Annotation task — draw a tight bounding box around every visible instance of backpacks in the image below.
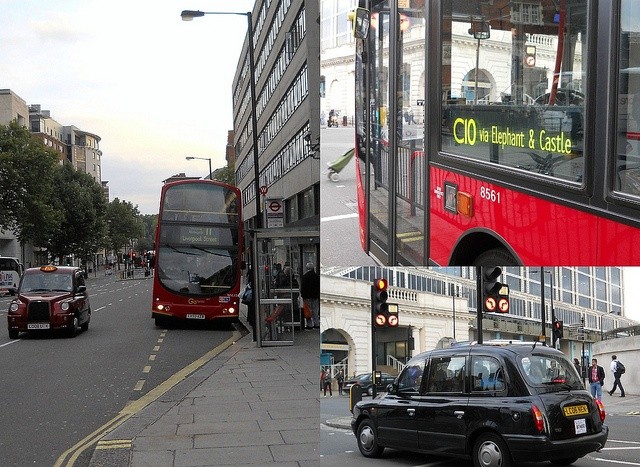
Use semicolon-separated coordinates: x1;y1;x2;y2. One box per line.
613;360;625;374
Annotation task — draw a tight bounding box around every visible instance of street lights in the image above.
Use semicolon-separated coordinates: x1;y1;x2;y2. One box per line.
185;155;213;183
180;10;259;219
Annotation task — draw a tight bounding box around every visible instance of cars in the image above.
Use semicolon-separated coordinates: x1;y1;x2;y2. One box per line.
343;371;397;393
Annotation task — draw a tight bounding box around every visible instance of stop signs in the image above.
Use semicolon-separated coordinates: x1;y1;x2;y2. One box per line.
268;199;285;214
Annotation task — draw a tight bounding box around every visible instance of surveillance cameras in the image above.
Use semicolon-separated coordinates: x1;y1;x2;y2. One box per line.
303;131;312;138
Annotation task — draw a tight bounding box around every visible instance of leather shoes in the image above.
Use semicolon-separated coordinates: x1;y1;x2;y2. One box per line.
619;395;625;397
607;390;611;395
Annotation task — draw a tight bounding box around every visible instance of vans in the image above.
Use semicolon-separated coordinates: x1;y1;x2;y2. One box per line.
0;255;23;292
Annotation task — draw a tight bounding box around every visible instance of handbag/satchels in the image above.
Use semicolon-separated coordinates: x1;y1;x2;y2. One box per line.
303;301;312;319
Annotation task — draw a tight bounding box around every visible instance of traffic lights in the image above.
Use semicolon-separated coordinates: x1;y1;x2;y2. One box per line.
555;320;563;338
370;278;390;313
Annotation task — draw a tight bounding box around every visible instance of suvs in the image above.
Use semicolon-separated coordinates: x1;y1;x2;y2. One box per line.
351;342;610;466
6;263;91;337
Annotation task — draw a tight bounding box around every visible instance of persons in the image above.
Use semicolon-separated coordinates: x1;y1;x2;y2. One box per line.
588;359;605;404
572;358;581;379
323;369;333;398
300;263;319;327
241;269;256;343
270;263;299;331
337;370;344;395
547;360;560;378
607;354;626;397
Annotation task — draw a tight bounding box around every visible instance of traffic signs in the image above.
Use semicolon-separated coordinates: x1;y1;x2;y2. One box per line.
372;313;399;327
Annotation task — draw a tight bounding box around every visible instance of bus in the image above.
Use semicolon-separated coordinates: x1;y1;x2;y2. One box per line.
151;176;244;325
347;1;640;264
